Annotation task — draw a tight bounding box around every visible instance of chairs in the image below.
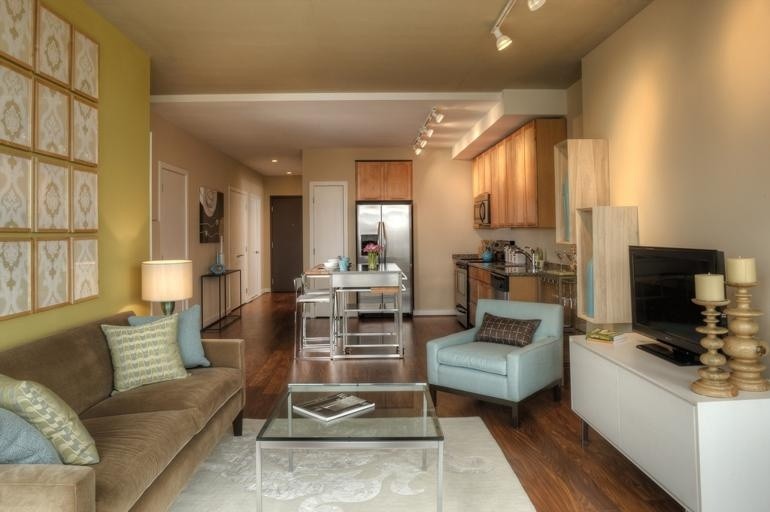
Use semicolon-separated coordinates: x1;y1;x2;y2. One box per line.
301;272;338;351
292;278;339;362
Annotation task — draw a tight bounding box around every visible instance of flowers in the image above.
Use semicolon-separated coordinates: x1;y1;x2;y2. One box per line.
362;242;385;269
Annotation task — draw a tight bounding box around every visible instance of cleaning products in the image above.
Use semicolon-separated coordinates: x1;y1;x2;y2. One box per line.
531;247;544;273
504;244;526;265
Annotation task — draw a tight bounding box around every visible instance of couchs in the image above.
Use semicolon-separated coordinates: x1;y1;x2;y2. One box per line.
425;295;567;427
0;311;248;512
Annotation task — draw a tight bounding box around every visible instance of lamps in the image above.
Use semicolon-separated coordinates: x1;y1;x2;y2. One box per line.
411;104;444;158
141;259;193;318
489;0;547;50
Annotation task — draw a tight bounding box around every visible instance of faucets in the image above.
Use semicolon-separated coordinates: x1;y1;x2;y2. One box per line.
513;247;531;263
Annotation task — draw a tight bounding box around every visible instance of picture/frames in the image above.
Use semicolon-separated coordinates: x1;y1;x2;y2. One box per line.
0;0;99;323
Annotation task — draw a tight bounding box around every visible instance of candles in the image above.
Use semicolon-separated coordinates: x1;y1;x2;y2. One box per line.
725;255;758;283
695;273;725;301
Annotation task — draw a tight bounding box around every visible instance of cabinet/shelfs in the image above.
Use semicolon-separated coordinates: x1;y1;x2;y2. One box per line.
466;264;490;325
567;328;770;512
471;116;567;229
355;160;413;203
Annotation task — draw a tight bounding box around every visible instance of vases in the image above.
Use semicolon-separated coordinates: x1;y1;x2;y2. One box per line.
368;252;377;269
218;236;226;271
210;249;224;274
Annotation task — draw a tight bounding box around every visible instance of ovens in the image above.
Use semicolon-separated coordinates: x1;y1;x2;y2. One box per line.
455;265;469;330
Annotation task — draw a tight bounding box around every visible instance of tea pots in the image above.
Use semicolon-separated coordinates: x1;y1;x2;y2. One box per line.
480;246;493;262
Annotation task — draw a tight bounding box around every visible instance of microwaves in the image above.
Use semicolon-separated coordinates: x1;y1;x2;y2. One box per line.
473;194;491;224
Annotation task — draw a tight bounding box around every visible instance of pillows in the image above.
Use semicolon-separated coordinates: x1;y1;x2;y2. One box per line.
101;312;191;398
474;308;542;348
1;408;64;467
126;302;210;368
1;370;101;466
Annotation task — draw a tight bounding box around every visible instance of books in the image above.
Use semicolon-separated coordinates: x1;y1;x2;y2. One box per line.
585;326;627;343
291;390;377;424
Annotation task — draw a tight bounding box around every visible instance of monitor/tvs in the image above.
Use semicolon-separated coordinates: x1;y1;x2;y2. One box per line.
629;246;728;367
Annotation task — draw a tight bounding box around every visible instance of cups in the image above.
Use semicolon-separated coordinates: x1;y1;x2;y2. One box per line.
323;255;350;271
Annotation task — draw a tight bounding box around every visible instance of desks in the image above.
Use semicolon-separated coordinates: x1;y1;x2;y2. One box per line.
200;268;242;332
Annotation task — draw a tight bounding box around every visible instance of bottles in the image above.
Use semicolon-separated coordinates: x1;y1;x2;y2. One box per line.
493;243;543;269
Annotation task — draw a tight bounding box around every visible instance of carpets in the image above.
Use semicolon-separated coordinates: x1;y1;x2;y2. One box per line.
172;414;536;511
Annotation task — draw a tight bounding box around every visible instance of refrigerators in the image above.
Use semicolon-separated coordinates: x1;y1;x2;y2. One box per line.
357;204;413;315
